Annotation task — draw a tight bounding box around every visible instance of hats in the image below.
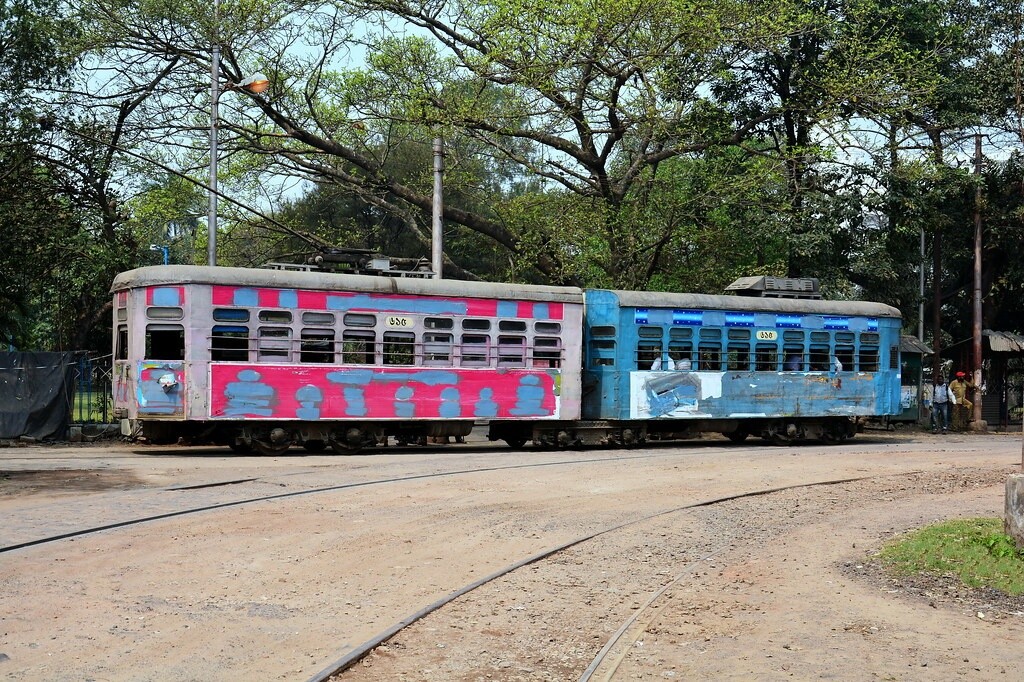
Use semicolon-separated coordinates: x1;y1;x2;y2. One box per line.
956;371;965;376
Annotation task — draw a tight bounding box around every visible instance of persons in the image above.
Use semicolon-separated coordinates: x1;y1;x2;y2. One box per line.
930;375;948;435
947;372;979;432
651;346;675;371
675;349;691;370
783;350;803;370
823;352;842;371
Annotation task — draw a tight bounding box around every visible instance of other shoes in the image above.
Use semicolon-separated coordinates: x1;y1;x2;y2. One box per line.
942;429;947;434
932;429;938;433
968;419;976;421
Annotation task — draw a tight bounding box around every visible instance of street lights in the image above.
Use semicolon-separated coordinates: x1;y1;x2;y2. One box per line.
208;42;271;265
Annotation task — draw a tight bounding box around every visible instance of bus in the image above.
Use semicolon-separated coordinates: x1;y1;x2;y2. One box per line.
36;117;902;457
